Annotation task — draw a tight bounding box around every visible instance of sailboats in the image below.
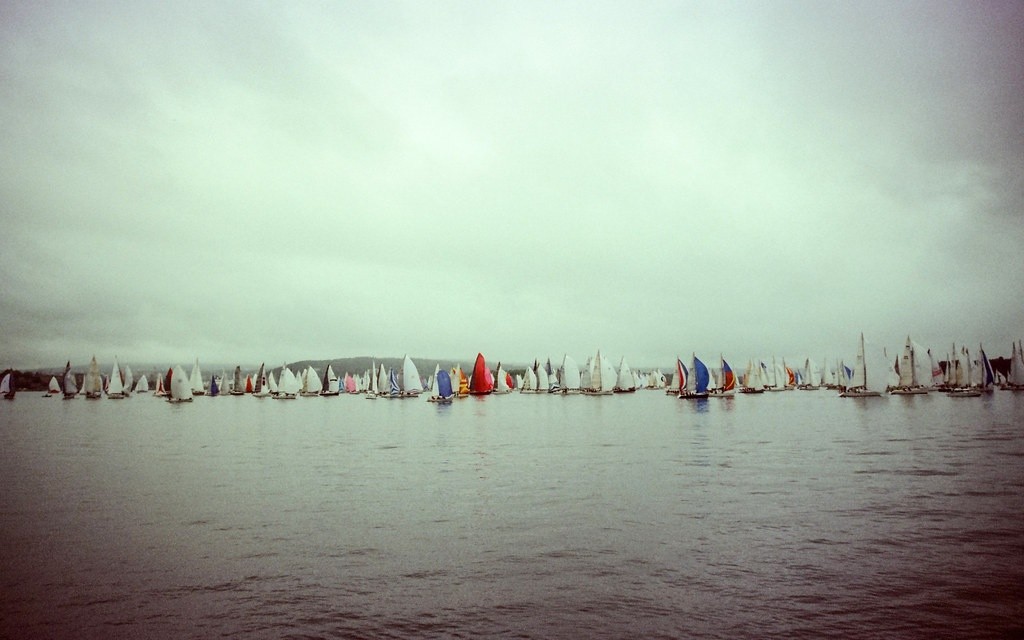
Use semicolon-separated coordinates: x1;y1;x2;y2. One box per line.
0;331;1024;412
